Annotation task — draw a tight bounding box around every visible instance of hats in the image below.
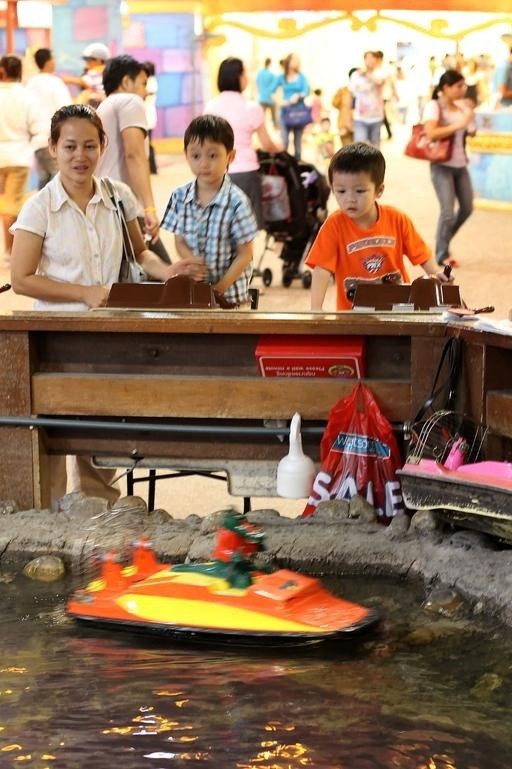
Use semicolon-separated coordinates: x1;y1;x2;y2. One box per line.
81;43;111;60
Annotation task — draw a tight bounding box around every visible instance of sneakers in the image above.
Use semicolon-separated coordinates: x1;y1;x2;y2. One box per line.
442;259;458;268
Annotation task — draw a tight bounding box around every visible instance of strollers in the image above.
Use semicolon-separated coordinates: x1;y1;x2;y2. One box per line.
248;143;333;290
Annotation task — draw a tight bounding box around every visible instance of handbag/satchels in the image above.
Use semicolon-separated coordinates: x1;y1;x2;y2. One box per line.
404;124;454;161
118;260;147;284
282;104;312;127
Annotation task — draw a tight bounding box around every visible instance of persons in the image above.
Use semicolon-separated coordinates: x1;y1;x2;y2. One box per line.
157;112;259;304
0;48;511;269
6;104;217;508
304;143;456;314
